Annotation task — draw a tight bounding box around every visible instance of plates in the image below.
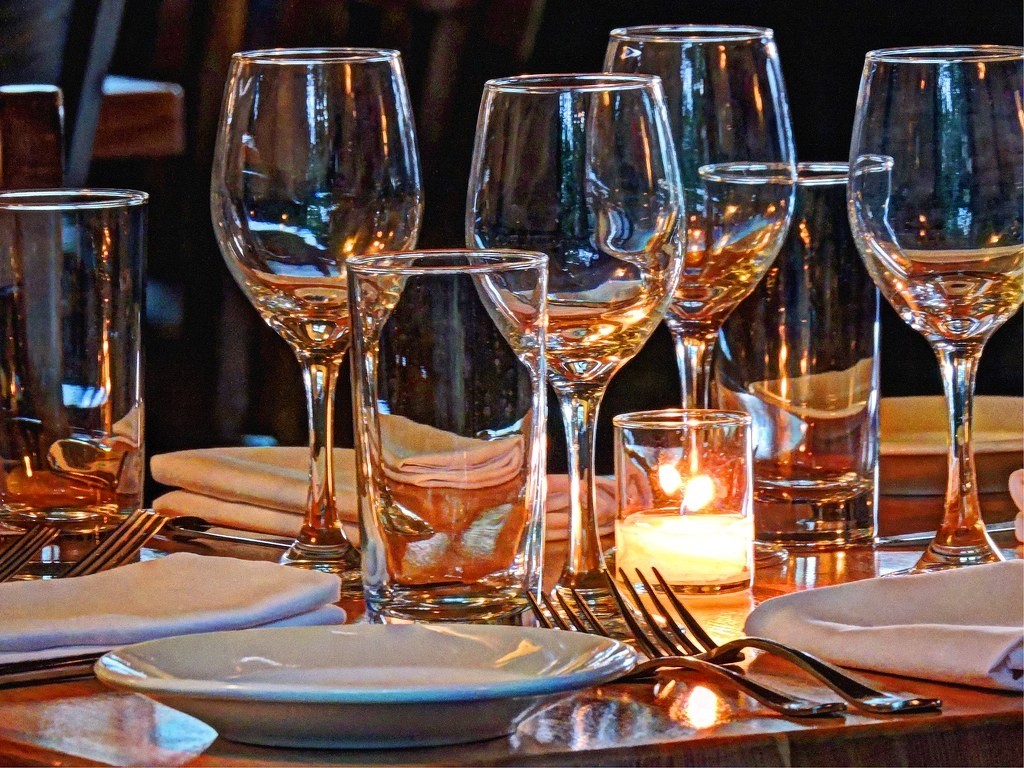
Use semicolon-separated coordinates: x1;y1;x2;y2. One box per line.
93;620;637;750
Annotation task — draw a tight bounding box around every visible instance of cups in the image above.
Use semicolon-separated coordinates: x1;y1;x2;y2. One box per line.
701;161;890;552
344;248;548;624
0;189;150;546
612;408;757;609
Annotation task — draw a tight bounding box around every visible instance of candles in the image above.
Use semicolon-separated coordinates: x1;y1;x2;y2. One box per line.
616;477;751;582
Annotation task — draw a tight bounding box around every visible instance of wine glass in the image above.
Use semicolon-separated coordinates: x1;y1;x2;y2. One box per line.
206;45;427;600
846;43;1024;578
585;18;797;404
462;73;687;615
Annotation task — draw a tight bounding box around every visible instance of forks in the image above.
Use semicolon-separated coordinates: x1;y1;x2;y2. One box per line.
602;565;943;714
526;586;848;714
63;508;170;579
0;523;61;584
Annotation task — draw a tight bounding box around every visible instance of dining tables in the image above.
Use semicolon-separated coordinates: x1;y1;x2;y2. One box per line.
1;495;1024;768
